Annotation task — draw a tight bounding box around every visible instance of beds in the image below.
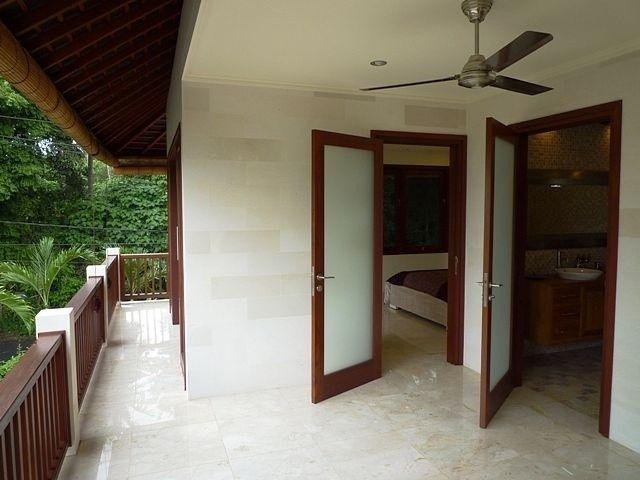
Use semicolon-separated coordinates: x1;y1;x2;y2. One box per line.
384;268;447;329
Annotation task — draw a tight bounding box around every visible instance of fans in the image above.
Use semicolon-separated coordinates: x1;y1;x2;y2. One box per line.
357;0;556;96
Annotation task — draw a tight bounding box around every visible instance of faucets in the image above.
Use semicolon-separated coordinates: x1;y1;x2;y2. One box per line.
556;250;565;267
576;257;593;267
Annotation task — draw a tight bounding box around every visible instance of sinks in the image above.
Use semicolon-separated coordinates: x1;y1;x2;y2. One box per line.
554;267;603;281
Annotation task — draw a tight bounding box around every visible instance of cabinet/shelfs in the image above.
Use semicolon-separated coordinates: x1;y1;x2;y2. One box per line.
582;284;603;340
549;287;580;341
